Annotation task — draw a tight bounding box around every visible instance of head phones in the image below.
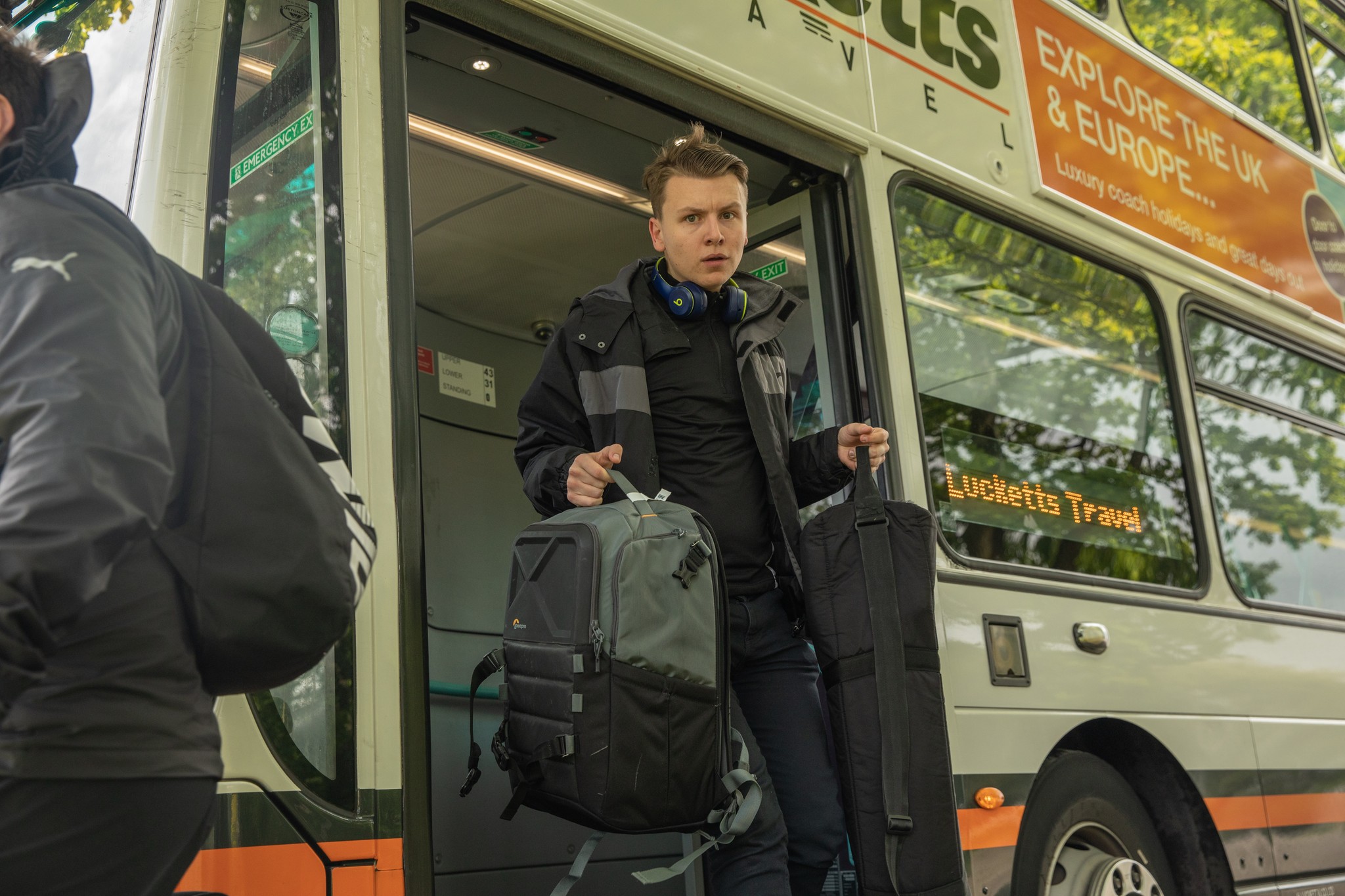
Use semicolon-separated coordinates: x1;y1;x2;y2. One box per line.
652;251;749;325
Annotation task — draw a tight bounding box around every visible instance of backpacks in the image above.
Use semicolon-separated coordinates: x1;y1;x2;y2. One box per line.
476;467;737;836
23;170;379;702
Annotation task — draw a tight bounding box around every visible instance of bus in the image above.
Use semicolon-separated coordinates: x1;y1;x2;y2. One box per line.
3;0;1345;896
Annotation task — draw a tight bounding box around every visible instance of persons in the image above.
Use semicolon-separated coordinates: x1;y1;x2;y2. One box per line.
0;25;225;896
515;121;891;896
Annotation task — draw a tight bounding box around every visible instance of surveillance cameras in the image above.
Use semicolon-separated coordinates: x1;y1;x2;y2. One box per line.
532;319;558;343
